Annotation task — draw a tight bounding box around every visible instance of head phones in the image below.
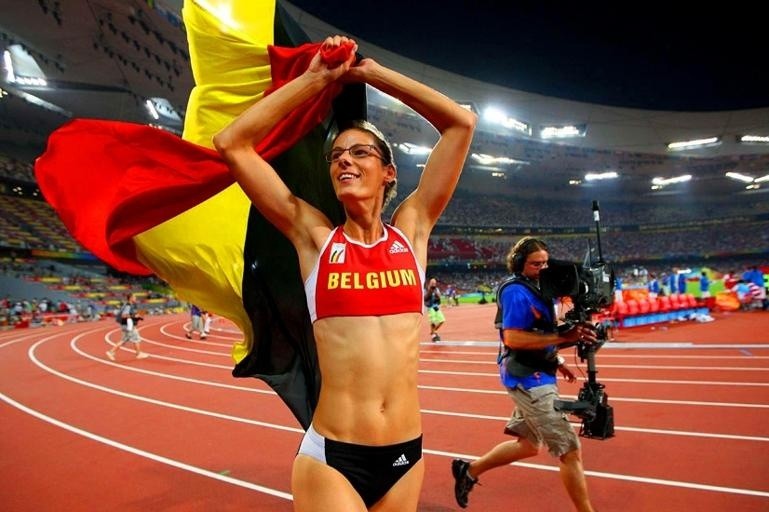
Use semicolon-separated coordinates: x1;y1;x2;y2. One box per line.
511;239;552;266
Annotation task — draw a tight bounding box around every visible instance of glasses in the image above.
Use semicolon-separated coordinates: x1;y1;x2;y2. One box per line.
326;142;386;159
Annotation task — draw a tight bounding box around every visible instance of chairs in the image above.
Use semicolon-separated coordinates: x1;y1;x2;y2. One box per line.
614;290;708;329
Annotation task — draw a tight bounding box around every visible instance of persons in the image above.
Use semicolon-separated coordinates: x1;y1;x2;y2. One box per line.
185;304;210;340
423;279;447;341
379;183;769;306
106;291;151;361
1;295;170;325
211;35;477;512
452;236;596;512
597;319;617;344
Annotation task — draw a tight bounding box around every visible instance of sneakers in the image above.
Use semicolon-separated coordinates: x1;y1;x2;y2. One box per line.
200;333;208;339
185;332;192;339
451;458;480;510
136;351;150;359
431;334;441;341
105;348;116;361
